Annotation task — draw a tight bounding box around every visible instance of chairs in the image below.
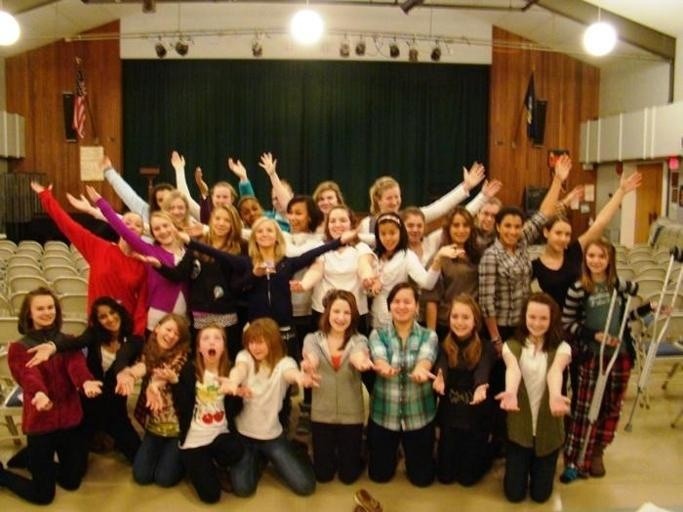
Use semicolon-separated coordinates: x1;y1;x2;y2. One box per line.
0;238;92;454
517;239;683;442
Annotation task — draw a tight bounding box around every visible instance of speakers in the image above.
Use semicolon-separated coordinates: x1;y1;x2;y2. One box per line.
63;94;77;142
525;186;548;245
527;100;547;146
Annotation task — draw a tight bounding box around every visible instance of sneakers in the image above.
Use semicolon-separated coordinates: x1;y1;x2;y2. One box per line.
591;456;604;476
8;446;29;469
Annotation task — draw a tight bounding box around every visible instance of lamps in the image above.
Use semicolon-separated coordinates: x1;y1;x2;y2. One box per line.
151;28;448;65
